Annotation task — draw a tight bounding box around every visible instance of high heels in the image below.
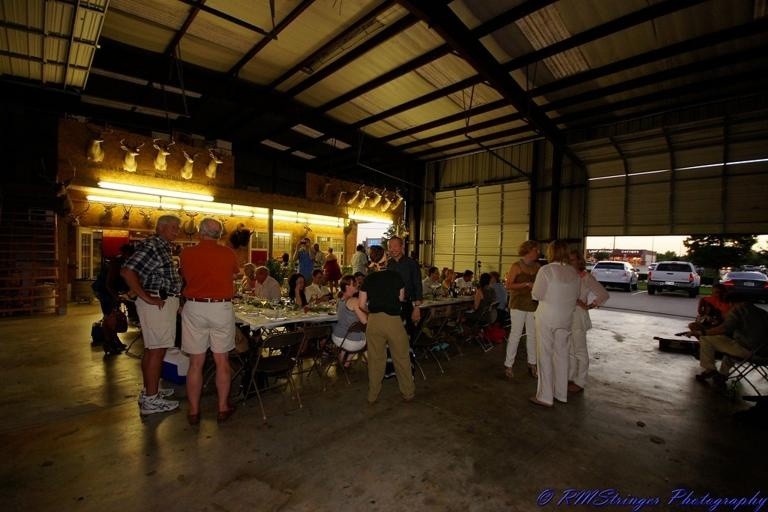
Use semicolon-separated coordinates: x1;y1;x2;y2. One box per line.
529;364;537;379
103;344;122;355
504;369;517;382
115;337;127;347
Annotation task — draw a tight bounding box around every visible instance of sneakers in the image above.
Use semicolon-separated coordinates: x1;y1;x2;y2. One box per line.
696;368;717;380
138;387;175;403
140;394;180;416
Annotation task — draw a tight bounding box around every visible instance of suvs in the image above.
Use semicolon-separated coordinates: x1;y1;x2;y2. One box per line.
647;261;705;300
591;260;640;291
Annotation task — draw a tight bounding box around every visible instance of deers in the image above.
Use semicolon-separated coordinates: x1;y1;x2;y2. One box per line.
205;149;224;179
180;144;199;180
119;137;146;173
320;175;332;195
82;127;114;163
152;137;175;171
333;183;404;213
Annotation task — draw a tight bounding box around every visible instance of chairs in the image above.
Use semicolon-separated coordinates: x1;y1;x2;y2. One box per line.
118;295;526;420
720;343;768;396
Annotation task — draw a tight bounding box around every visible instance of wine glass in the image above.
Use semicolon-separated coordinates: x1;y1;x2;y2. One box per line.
233;295;338;321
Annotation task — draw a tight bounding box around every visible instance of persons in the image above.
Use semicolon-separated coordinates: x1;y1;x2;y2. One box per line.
118;214;185;418
684;283;767;381
530;236;582;409
357;244;418;406
178;217;241;426
567;247;611;394
89;235;511;384
501;240;543;379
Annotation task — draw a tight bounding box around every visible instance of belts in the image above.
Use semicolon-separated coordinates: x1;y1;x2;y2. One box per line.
148;290;181;298
188;298;231;303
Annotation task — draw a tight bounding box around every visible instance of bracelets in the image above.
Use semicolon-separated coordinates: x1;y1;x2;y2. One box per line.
589;303;593;309
525;281;530;286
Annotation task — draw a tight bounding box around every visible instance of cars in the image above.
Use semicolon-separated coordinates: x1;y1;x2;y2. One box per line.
712;272;767;306
648;261;657;270
586;261;595;270
721;264;766;272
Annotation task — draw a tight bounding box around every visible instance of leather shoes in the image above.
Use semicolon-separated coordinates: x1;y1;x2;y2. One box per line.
528;384;585;407
186;411;200;425
216;407;236;424
